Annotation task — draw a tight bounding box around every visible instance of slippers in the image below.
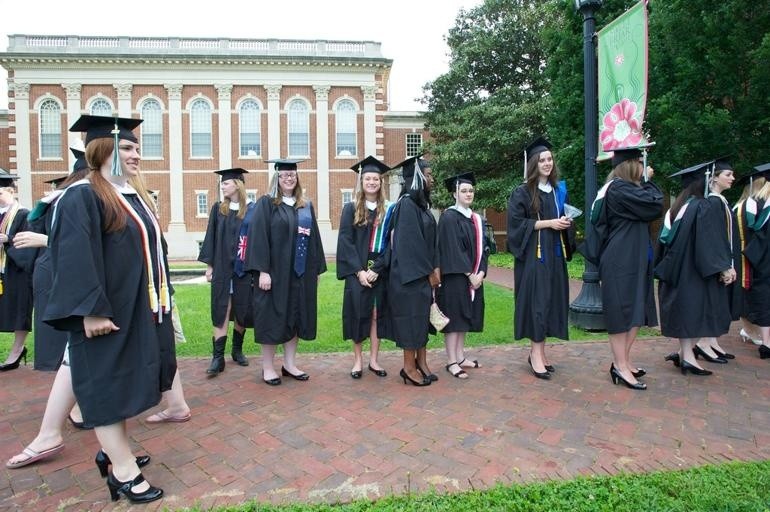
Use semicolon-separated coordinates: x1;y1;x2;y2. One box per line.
6;444;65;469
145;412;191;423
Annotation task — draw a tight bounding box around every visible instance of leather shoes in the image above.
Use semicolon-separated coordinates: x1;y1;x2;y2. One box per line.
68;415;94;429
369;363;387;377
282;366;310;380
350;371;362;379
528;355;554;379
262;369;281;385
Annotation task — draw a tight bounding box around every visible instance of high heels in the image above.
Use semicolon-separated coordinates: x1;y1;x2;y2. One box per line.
0;346;28;371
400;360;437;386
758;345;770;359
664;344;735;375
740;329;762;345
106;470;163;503
610;362;648;390
95;450;150;478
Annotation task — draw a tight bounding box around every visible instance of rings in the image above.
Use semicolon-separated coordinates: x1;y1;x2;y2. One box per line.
438;284;443;289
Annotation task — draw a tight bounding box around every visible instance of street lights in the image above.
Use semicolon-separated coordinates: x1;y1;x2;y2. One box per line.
569;0;652;333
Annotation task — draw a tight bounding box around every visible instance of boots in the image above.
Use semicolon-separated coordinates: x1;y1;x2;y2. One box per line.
206;335;227;375
231;328;250;366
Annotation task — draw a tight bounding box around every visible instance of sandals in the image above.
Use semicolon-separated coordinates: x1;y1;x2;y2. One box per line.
458;358;483;368
445;362;469;379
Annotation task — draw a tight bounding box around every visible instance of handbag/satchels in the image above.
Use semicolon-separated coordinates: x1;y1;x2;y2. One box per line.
430;304;450;331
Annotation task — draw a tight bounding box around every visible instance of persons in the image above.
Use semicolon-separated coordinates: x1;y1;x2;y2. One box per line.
376;165;444;387
244;170;329;387
198;179;256;376
505;150;574;380
588;146;770;392
437;183;489;380
1;115;193;505
336;172;394;380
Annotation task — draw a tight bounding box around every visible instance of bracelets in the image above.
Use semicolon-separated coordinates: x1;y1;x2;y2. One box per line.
538;221;541;230
467;272;474;279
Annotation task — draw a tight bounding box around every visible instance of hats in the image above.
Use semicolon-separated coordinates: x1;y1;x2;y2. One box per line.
69;114;144;177
349;151;477;210
518;136;554;179
667;153;769;199
603;142;654;184
70;148;87;169
44;176;68;187
0;175;21;188
214;167;249;183
263;158;305;198
